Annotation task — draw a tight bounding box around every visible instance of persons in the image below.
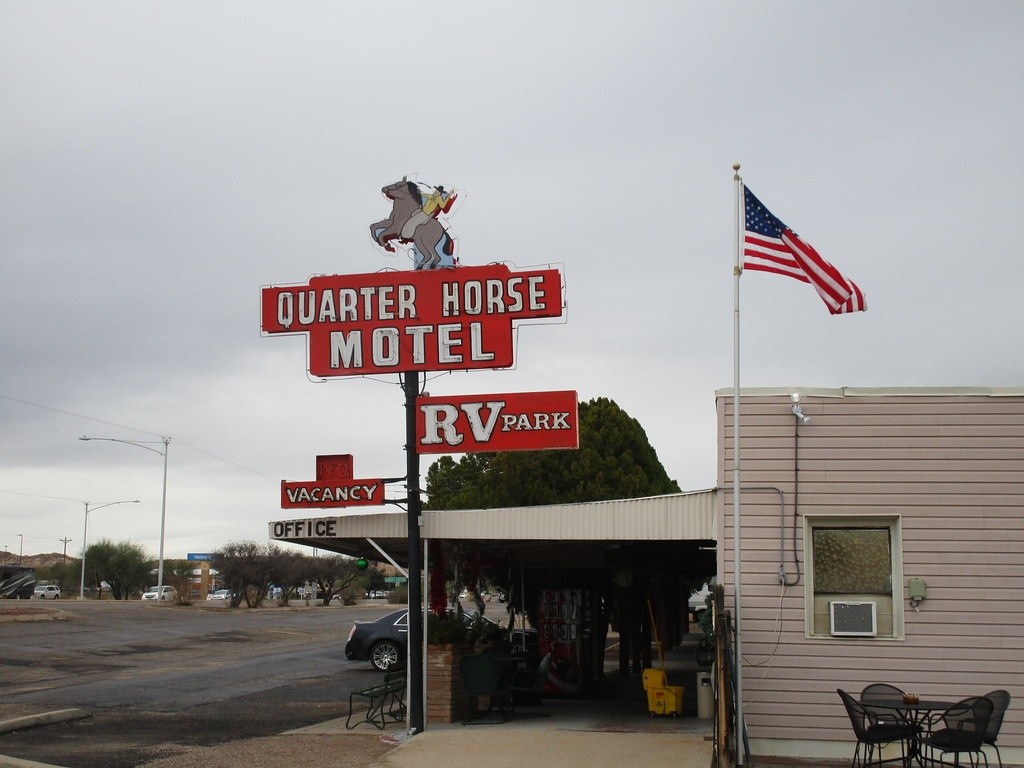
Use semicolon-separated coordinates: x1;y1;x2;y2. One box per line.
615;591;644;676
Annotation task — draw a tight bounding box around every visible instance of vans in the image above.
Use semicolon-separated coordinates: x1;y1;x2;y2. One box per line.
34;585;60;600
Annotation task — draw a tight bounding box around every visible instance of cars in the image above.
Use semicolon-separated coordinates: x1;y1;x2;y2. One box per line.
210;590;234;599
366;590;390;599
333;595;342;600
141;585;178;601
344;605;541;673
458;593;467;598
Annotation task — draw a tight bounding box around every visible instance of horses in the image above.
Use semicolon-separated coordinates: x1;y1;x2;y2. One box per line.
368;175;454;270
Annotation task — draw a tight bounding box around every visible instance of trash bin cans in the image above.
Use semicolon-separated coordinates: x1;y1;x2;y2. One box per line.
695;670;715;720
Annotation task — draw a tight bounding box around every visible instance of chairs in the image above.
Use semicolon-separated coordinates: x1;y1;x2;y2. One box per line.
836;684;1011;768
459;653;508;724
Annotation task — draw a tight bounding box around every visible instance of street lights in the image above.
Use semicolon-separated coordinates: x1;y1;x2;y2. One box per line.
18;533;23;565
4;545;8;564
79;434;171;606
80;499;140;601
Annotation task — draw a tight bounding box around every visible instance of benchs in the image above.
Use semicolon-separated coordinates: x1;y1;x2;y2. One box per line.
346;660;407;730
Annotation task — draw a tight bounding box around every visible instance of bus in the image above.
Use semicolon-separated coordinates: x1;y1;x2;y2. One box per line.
0;564;37;600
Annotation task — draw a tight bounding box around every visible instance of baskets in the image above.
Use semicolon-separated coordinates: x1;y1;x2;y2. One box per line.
903;694;919;705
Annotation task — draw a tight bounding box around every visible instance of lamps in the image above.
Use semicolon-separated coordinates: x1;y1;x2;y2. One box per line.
791;393;811;425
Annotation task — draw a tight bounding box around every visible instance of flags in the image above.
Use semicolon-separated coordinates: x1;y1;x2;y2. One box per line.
740;183;869;316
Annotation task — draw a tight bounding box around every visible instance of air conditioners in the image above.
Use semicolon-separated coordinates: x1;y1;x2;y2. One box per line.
828;601;877;637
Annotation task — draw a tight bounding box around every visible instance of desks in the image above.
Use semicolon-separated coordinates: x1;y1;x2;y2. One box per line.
858;700;970;768
470;656;527;716
